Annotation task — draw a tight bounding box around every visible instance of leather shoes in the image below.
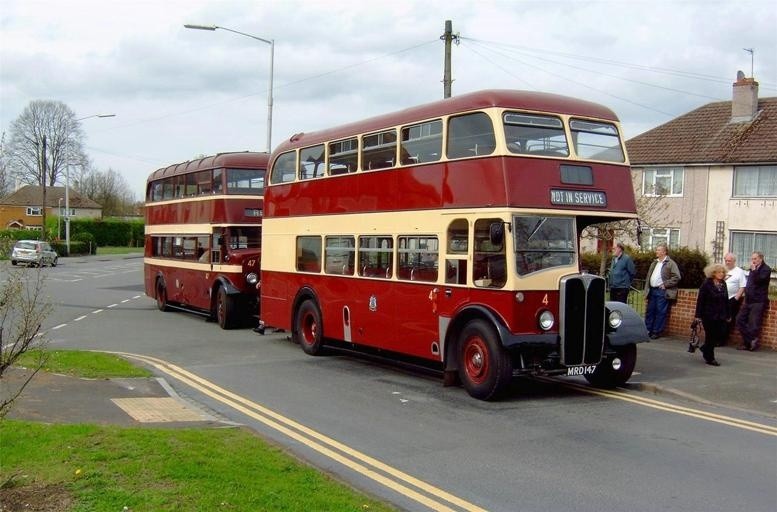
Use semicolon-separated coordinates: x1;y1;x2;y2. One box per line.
647;330;659;339
736;337;760;351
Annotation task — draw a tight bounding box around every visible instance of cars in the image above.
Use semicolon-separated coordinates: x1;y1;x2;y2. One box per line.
11;240;58;268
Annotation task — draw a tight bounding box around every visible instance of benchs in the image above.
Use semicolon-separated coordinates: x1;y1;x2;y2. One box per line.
320;137;495;177
300;253;459;283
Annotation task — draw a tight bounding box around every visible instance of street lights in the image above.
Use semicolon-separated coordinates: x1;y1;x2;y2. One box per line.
57;198;63;240
66;114;115;257
184;23;274;154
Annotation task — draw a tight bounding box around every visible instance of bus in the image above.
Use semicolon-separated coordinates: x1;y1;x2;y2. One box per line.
259;87;652;401
143;152;319;330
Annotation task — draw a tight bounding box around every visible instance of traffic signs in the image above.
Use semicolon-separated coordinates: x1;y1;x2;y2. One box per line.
26;207;42;216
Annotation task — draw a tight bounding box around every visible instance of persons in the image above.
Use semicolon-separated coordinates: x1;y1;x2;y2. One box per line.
688;263;730;369
251;279;287;335
642;245;682;341
735;252;769;352
716;252;747;347
607;241;636;302
